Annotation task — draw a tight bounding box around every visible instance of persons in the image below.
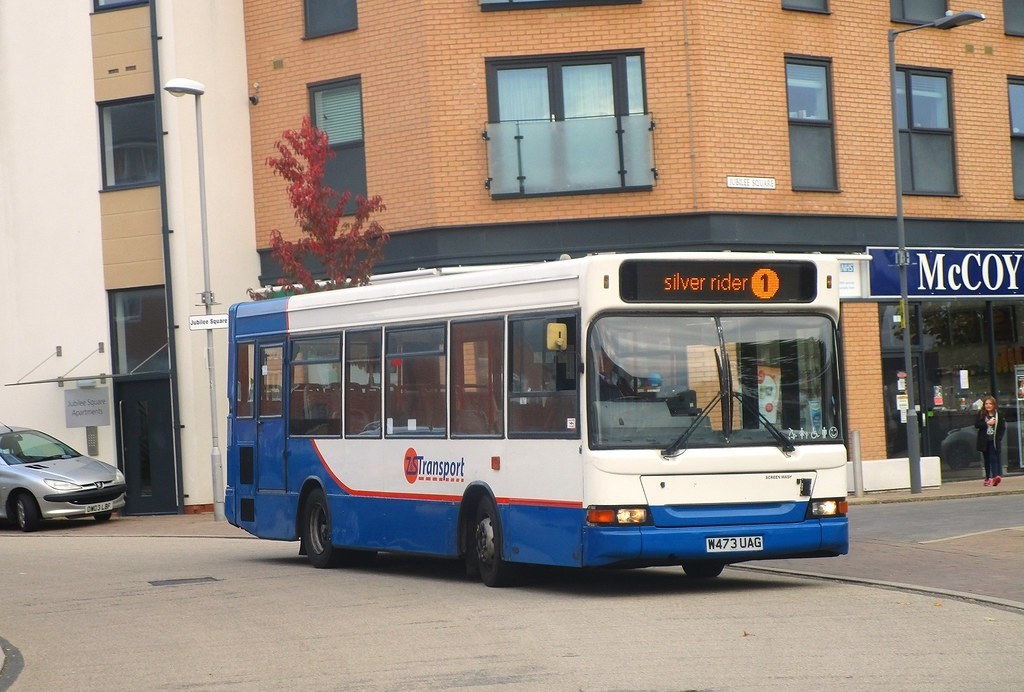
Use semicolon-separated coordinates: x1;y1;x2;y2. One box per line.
595;341;633;402
975;395;1006;486
316;407;340;435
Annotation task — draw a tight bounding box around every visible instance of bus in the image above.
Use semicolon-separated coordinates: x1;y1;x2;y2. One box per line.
224;254;849;585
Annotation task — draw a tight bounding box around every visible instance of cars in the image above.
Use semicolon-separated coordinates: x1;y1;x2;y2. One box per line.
939;404;1024;471
0;426;127;532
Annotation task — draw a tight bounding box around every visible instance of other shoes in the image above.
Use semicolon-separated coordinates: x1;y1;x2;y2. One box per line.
991;475;1001;486
984;478;991;486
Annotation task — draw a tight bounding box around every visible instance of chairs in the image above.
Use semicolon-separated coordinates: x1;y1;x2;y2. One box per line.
253;382;501;436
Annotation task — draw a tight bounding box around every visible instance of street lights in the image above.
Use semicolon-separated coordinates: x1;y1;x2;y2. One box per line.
163;77;227;522
888;10;986;497
